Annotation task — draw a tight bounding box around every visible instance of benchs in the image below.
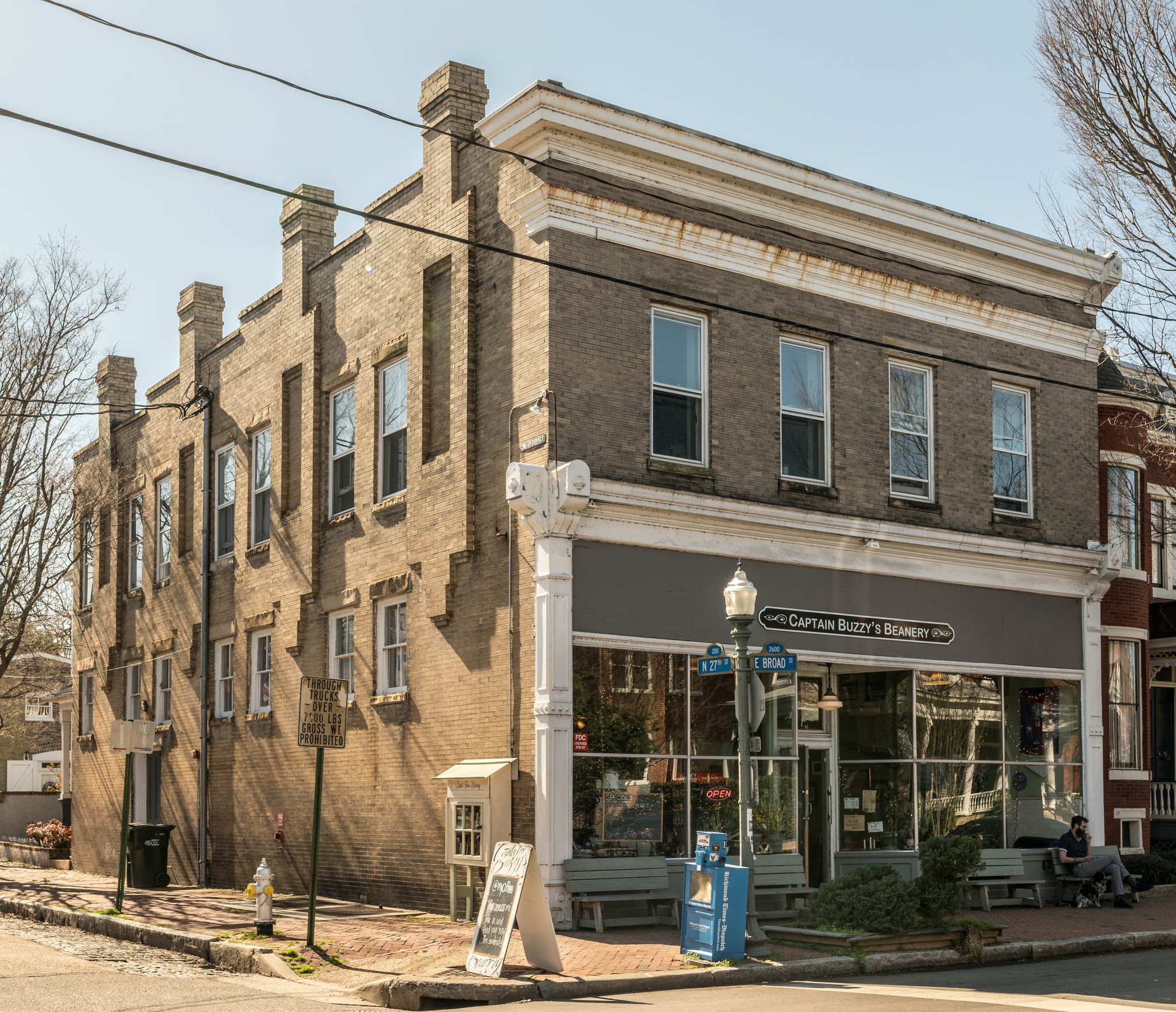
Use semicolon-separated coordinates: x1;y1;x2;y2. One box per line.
955;849;1046;912
1051;846;1143;908
563;855;683;934
725;853;818;920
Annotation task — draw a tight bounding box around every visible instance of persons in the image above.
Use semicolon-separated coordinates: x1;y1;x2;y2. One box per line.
1057;814;1155;911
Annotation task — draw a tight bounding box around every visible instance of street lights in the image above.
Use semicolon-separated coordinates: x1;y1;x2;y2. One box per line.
724;557;771;958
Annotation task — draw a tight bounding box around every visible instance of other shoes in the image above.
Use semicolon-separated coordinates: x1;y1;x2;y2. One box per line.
1131;884;1154;893
1113;899;1133;909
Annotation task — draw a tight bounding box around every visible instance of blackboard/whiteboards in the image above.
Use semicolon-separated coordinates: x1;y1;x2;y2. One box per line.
472;873;520;959
601;788;664;843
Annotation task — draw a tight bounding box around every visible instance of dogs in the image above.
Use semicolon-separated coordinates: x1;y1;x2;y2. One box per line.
1054;871;1109;908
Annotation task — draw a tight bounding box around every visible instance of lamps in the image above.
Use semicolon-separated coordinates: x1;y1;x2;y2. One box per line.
530;389;549;413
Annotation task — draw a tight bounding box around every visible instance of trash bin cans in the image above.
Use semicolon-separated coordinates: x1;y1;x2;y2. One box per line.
120;822;176;889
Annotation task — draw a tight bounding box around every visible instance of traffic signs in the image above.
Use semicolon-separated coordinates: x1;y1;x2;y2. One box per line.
298;676;349;748
750;640;798;673
697;643;735;676
